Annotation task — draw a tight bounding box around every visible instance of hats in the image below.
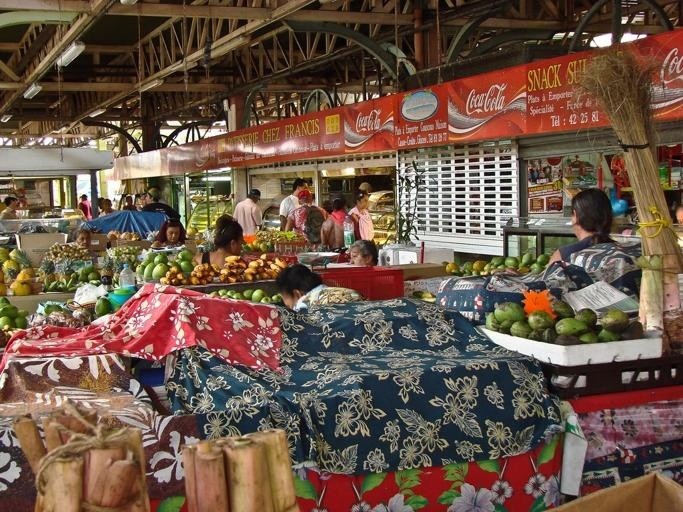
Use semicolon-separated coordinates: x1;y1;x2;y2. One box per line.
250;188;262;201
298;190;312;200
144;186;162;199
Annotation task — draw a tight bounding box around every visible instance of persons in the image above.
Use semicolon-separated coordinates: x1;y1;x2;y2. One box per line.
80;187;181;222
194;216;242;271
278;178;374;255
72;230;98;264
0;197;19;219
233;189;262;234
348;239;379;268
157;218;187;245
549;187;642;302
275;264;367;313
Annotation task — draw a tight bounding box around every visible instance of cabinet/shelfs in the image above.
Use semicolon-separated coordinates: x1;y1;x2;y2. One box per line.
259;203;282;231
364;190;396;247
184;196;233;237
501;214;577;268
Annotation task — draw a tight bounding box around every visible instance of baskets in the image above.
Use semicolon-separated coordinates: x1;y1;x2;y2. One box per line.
67;301;96;314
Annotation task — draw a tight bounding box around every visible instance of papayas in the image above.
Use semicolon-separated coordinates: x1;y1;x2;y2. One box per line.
78;264;101;287
43;304;63;315
108;245;141;267
159;254;289;286
485;297;644;346
136;250;198;281
446;252;550;276
241;240;272;252
95;298;112;316
0;297;29;330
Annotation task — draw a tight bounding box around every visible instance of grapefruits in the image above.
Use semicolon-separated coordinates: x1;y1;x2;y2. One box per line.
10;280;31;296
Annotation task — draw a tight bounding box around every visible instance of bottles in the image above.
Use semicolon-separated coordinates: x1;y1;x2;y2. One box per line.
343;216;357;245
118;263;135;292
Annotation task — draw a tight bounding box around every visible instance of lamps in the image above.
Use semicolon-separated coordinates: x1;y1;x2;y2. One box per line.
0;110;15;124
23;81;45;99
56;39;86;69
87;105;107;118
139;77;166;94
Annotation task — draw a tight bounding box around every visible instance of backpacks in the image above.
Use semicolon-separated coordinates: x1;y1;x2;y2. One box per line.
302;205;326;244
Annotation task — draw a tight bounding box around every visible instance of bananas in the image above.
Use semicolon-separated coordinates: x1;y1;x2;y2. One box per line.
44;276;76;292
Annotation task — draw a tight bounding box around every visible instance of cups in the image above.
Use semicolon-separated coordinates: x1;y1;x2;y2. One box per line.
274;242;330;254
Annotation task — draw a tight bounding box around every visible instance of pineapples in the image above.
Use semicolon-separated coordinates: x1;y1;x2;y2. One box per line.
0;248;75;284
101;257;123;288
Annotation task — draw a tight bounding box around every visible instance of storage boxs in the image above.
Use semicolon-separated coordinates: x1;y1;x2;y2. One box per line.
377;245;416;268
11;230;67;269
477;323;664;387
542;469;682;511
398;245;456;269
85;233;107;253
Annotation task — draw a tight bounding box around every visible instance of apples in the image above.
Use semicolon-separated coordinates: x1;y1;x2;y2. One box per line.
211;289;282;304
